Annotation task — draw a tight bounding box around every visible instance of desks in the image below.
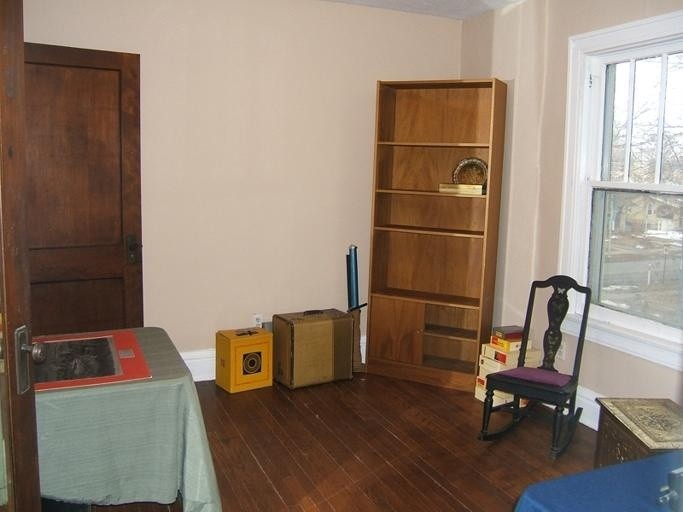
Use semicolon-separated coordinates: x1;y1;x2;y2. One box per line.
35;326;224;512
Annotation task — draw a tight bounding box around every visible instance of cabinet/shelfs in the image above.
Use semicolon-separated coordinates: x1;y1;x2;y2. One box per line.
594;398;683;468
362;76;508;393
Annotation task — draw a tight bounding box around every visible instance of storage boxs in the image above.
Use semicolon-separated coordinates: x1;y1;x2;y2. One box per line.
272;308;355;390
475;325;544;408
212;326;272;394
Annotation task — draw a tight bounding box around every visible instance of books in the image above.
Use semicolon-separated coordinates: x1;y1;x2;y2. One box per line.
438;183;486;195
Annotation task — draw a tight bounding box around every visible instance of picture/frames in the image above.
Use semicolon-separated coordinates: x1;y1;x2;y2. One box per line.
30;330;152;392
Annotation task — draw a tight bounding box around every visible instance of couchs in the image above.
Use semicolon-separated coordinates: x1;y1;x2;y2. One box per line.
513;449;683;512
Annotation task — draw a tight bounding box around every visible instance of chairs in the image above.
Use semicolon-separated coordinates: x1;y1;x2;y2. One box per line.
478;275;591;461
